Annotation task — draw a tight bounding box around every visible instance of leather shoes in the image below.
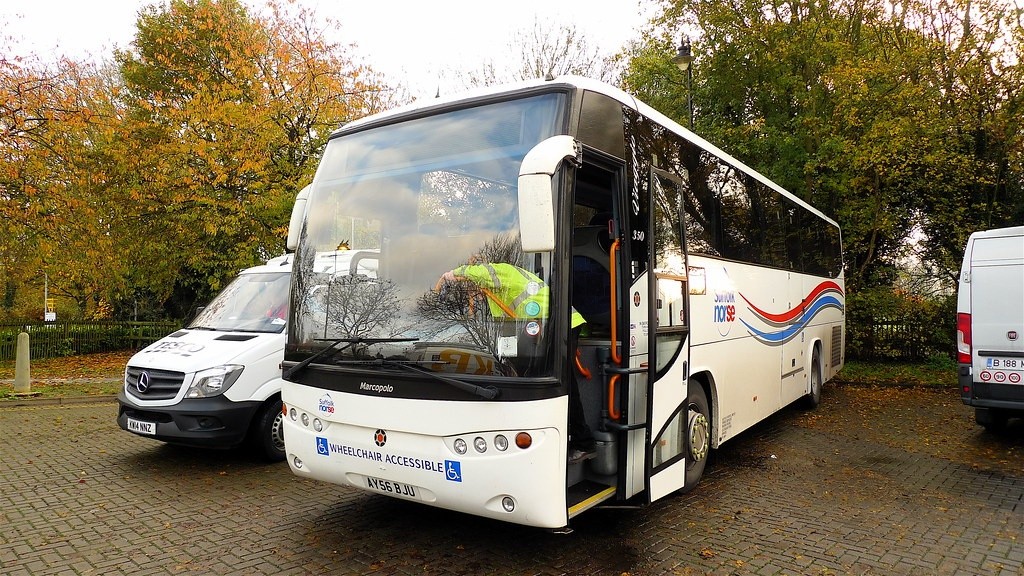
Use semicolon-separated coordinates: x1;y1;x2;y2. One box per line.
568;450;597;464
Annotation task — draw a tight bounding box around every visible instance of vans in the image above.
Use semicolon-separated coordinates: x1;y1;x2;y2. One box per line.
116;247;380;459
956;225;1024;436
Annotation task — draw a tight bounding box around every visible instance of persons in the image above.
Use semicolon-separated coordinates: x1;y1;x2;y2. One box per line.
442;263;598;463
267;296;287;320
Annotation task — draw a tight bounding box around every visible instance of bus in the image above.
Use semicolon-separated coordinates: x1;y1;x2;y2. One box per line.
278;74;847;530
